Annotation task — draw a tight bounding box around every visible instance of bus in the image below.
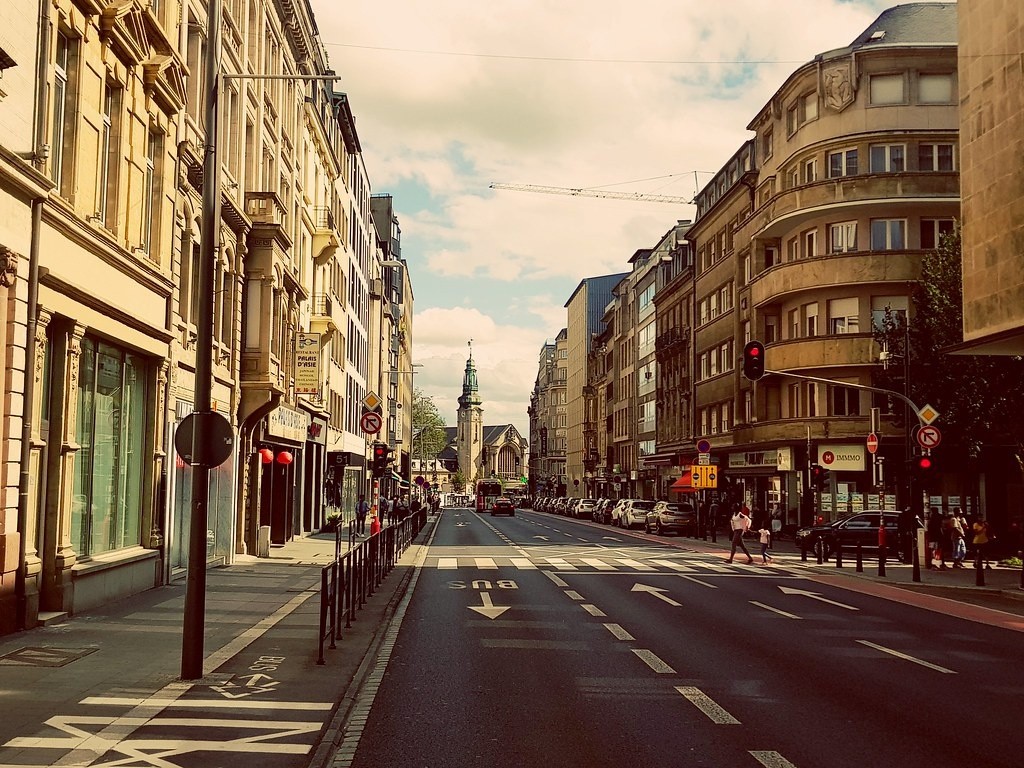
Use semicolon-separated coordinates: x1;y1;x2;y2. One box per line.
474;479;502;513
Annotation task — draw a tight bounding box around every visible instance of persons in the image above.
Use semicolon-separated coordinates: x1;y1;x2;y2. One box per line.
929;506;993;569
750;521;773;565
742;501;749;516
427;493;432;512
771;504;782;540
724;503;753;564
650;495;671;502
709;500;718;518
355;495;371;538
410;496;421;514
380;492;409;528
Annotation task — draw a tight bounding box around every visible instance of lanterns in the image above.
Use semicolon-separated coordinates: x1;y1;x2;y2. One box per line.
277;451;293;464
260;449;273;463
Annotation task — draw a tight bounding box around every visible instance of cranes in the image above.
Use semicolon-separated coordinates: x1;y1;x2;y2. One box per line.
488;170;716;204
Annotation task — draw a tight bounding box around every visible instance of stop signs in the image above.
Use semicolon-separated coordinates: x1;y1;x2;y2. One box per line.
816;514;824;522
866;433;878;454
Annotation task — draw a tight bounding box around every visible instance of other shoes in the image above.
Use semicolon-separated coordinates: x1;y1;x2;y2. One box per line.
762;562;768;565
355;533;360;537
361;533;365;537
953;564;963;569
724;560;732;563
747;559;753;565
769;557;773;564
973;563;977;568
986;565;991;570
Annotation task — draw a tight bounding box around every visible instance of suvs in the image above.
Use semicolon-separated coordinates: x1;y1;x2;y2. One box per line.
490;498;515;516
794;510;912;565
644;500;696;537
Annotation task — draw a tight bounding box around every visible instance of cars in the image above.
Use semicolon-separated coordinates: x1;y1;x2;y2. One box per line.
512;496;658;530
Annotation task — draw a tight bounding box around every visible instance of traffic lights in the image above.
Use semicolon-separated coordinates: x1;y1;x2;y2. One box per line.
749;345;760;376
919;455;934;471
385;447;396;464
821;468;830;489
375;444;385;473
811;466;823;484
492;470;495;476
515;457;519;465
434;487;437;492
427;488;431;493
